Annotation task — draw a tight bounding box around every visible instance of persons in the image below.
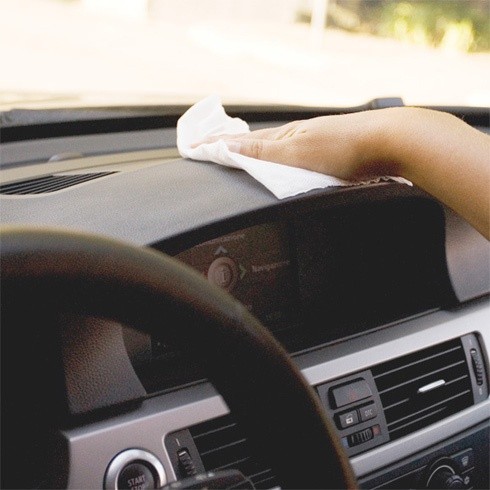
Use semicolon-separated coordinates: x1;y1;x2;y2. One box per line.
191;106;489;245
